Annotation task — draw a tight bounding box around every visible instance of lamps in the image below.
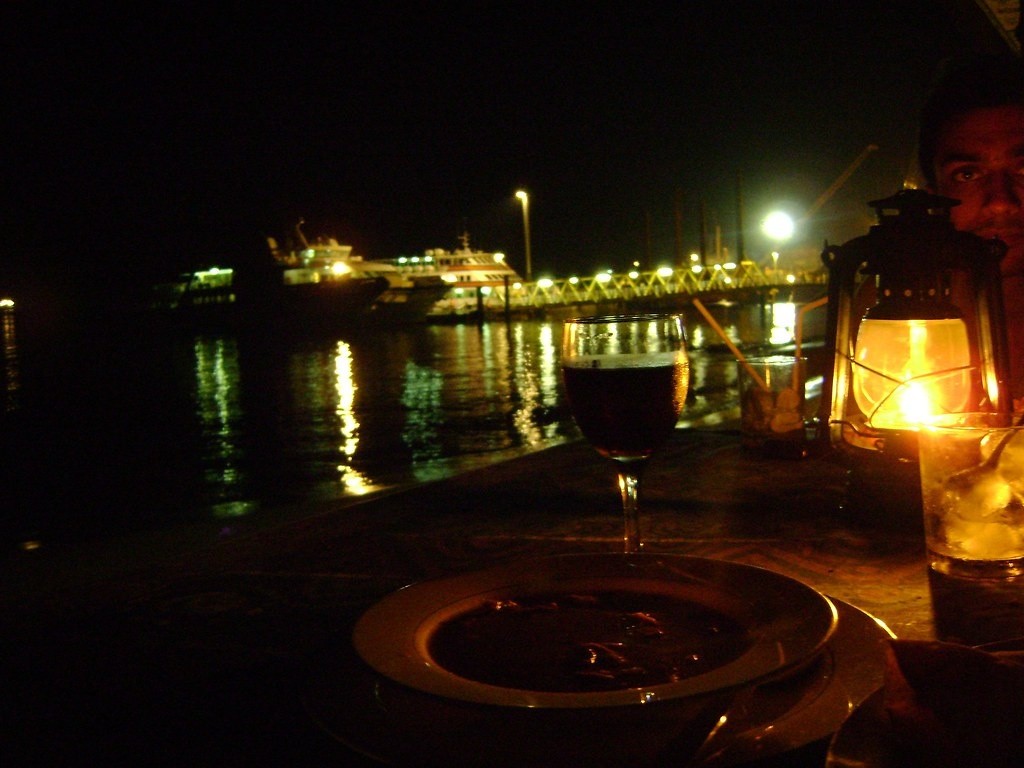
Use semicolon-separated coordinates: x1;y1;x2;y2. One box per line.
816;187;1013;542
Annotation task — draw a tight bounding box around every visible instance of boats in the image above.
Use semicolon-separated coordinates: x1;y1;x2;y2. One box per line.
171;220;524;336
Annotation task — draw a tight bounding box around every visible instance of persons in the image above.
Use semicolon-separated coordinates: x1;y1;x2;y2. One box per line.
918;47;1024;428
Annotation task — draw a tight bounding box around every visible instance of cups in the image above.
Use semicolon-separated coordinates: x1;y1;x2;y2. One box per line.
916;412;1024;648
736;356;809;456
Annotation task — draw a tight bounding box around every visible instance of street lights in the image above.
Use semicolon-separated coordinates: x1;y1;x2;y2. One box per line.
516;190;532;275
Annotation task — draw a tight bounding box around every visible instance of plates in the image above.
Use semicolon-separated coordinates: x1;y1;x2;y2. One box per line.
301;590;898;768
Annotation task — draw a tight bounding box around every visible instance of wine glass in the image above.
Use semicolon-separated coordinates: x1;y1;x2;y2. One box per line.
562;313;692;555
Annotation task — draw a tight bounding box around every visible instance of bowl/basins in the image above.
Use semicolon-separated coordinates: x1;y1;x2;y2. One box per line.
353;556;838;719
